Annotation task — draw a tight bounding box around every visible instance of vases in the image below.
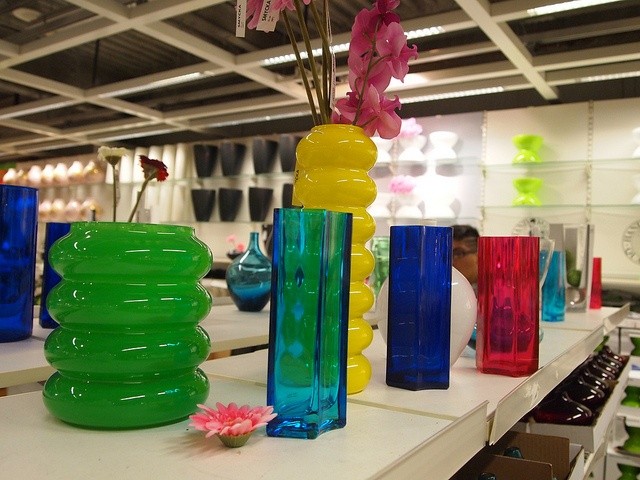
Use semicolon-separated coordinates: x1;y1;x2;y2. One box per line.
42;221;213;430
228;253;243;260
225;231;273;312
290;125;378;395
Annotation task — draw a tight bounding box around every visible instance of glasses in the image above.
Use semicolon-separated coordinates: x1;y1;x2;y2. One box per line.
453;247;477;258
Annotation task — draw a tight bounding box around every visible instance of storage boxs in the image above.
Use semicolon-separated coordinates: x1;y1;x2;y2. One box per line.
482;429;582;480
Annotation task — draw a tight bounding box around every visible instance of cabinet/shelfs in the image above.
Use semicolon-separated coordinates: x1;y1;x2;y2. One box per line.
173;170;303;271
3;180;97;225
480;155;639;301
372;156;483;223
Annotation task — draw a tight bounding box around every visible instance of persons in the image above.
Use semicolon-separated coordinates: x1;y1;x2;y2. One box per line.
450;226;480;286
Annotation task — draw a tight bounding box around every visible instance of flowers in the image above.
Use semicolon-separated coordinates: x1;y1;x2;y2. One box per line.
96;180;169;223
191;402;278;439
238;0;419;139
224;236;245;252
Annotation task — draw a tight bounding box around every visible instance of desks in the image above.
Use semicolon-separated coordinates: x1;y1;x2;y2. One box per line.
546;300;634;329
0;337;55;390
198;308;605;445
0;378;492;480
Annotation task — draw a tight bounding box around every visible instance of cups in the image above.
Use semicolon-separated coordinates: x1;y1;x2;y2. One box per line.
476;234;542;376
265;208;355;440
0;185;40;343
385;224;453;393
